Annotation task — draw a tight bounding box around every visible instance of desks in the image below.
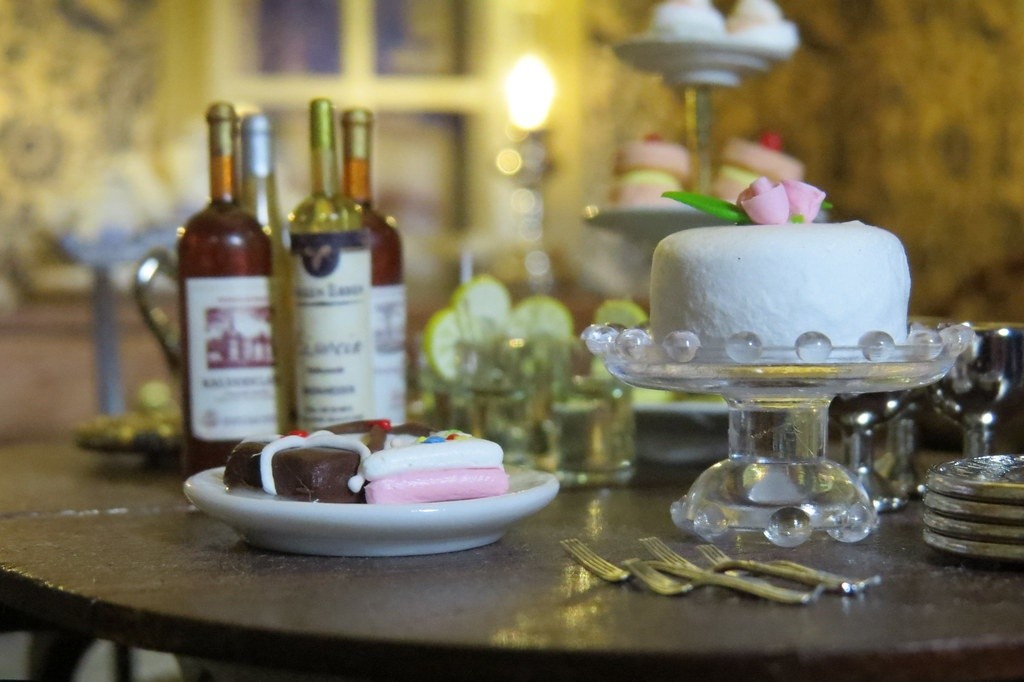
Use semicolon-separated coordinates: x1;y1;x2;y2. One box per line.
0;441;1024;682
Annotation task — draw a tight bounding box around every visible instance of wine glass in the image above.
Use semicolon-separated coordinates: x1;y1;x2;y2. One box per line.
828;322;1024;509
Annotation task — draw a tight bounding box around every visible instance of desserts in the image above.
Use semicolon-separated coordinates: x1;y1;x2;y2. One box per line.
608;130;815;208
221;416;510;505
642;0;800;58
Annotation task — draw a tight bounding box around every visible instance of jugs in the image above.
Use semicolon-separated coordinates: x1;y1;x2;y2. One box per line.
138;228;186;460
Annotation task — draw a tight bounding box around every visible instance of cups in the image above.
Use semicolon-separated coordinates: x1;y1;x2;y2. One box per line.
421;324;638;486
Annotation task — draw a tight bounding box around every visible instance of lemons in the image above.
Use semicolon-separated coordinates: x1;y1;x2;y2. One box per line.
422;274;652;382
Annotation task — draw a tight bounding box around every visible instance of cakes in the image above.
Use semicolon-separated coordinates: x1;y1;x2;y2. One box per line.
647;179;911;363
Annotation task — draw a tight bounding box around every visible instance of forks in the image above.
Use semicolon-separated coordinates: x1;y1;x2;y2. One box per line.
559;536;884;611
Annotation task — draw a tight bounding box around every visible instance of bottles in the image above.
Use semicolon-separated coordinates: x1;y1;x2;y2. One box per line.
285;96;376;430
339;104;407;431
174;102;281;481
239;108;293;433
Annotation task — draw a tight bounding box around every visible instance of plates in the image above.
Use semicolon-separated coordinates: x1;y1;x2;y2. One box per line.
71;426;185;457
919;450;1024;558
183;466;560;556
